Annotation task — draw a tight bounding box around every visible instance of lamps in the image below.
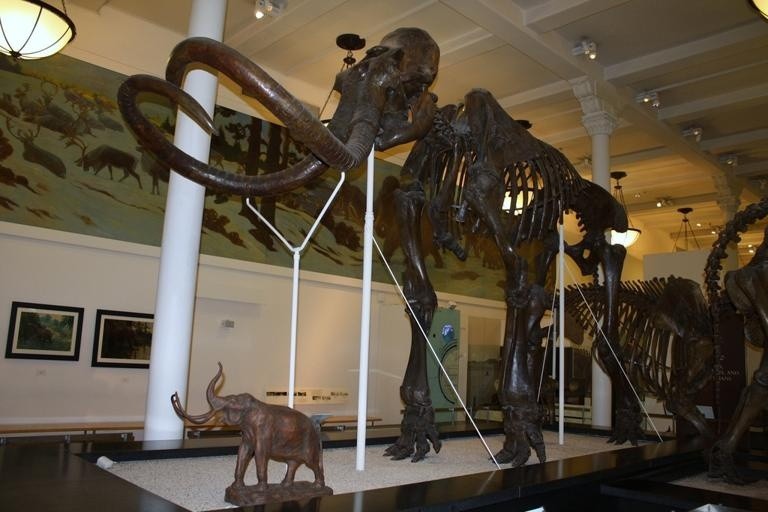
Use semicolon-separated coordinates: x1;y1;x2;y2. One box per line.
610;172;642;247
0;0;75;60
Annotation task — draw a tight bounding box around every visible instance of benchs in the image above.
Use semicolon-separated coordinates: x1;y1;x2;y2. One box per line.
0;416;383;443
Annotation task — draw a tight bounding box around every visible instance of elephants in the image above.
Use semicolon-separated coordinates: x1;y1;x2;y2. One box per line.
169;360;331;490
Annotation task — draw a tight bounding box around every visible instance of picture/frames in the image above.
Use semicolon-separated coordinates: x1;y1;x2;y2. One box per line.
5;301;84;361
92;309;154;368
